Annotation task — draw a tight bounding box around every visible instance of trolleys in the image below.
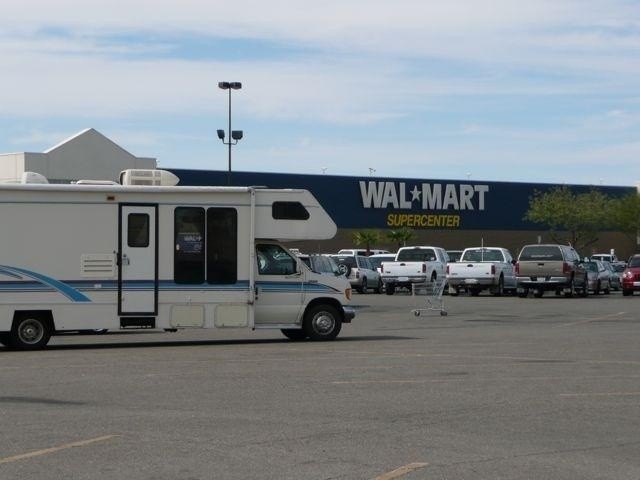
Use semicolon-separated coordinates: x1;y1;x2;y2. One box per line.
412;281;450;316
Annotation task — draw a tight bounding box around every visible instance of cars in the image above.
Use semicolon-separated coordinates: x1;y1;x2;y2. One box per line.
586;252;625;294
622;254;640;298
333;251;384;296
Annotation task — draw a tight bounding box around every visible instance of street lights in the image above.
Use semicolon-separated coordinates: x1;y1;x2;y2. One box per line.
218;81;243;171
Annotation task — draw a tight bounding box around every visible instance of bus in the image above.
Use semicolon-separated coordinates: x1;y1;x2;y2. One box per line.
1;167;356;350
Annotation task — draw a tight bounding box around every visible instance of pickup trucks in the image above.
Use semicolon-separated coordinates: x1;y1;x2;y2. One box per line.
446;245;516;296
382;246;449;296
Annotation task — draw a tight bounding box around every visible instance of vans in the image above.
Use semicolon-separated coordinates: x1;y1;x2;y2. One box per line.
515;243;591;296
